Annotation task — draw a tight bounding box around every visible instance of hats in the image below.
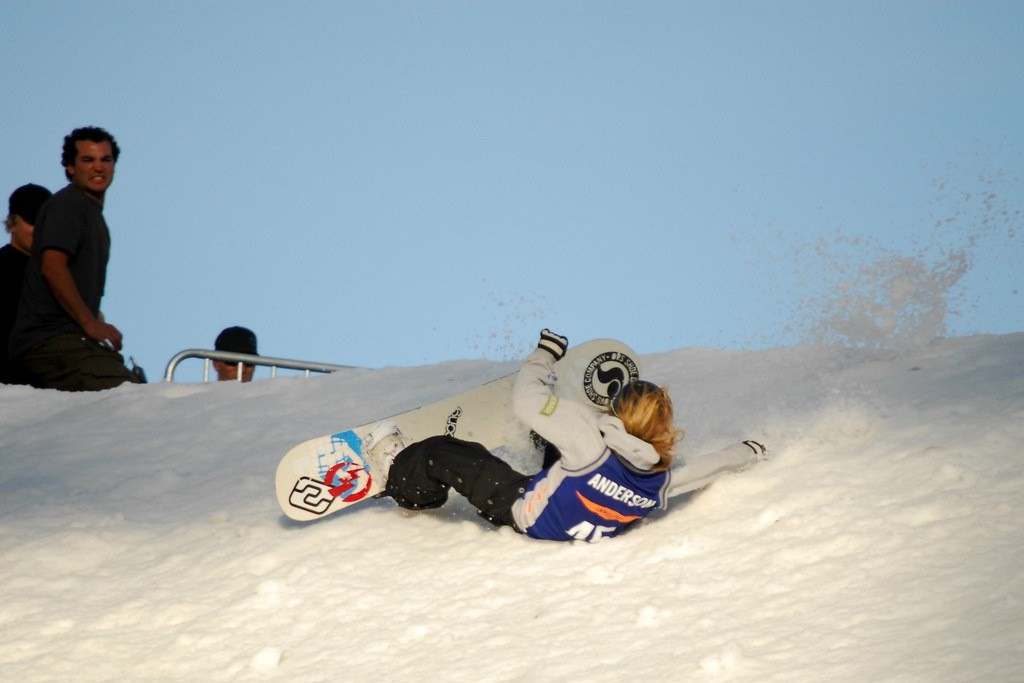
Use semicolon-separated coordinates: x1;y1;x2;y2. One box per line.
8;183;53;225
215;325;259;357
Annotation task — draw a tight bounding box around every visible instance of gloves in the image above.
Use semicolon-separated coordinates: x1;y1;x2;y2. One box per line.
538;328;568;360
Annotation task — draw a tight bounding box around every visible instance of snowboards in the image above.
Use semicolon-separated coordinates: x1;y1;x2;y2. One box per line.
274;337;642;523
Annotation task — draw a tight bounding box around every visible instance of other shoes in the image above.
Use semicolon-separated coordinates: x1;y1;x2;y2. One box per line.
370;428;405;478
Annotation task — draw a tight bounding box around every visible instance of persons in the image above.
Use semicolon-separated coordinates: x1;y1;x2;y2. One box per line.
0;183;54;386
26;124;137;392
212;326;259;382
364;327;682;544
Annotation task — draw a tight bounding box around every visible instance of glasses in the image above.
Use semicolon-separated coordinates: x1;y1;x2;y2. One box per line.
224;361;253;367
